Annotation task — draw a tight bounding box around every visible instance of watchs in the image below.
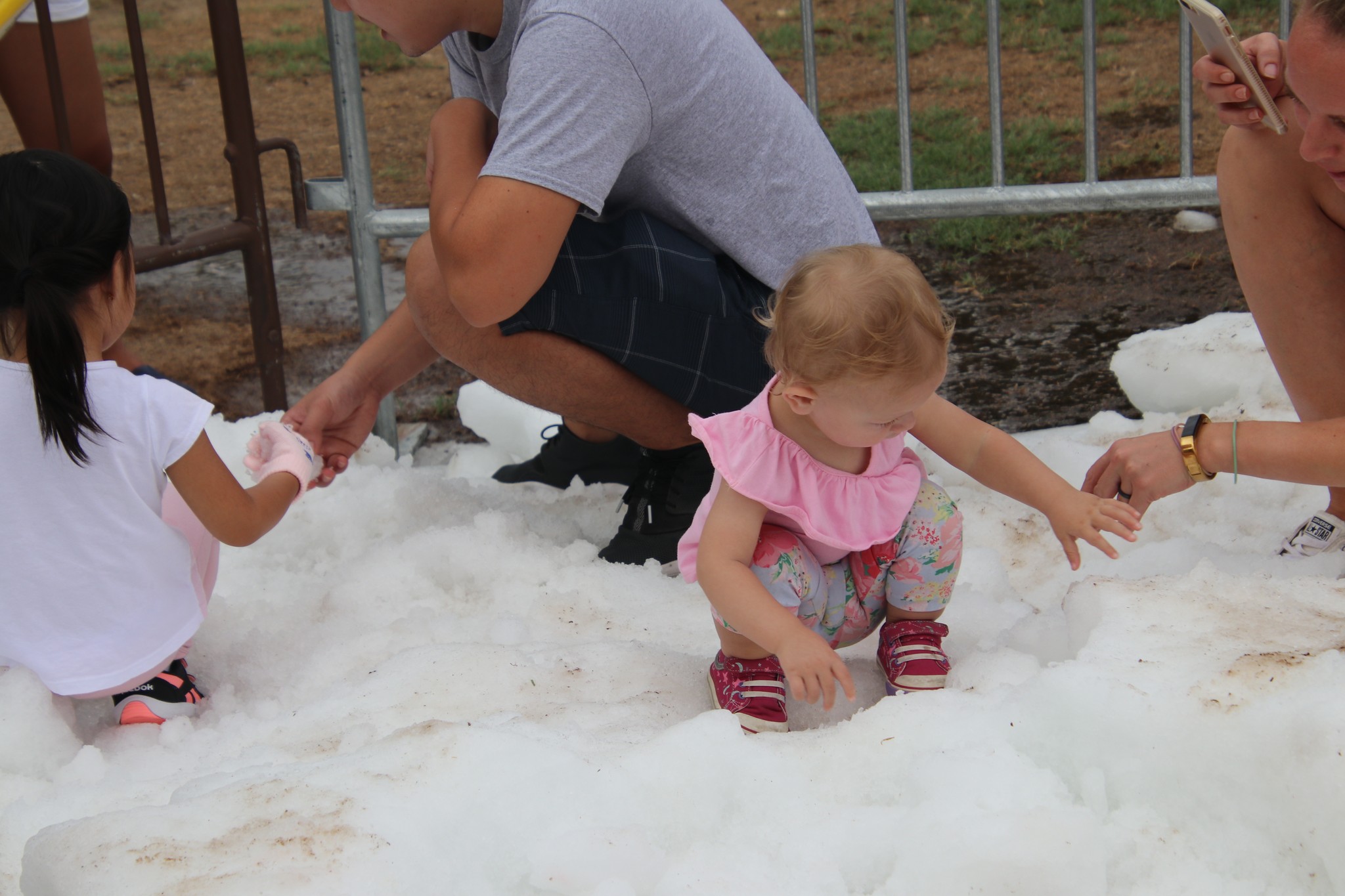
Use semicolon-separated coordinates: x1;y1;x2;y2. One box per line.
1179;413;1220;483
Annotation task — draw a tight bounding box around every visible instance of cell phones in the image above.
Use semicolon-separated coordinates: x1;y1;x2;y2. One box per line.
1176;1;1289;135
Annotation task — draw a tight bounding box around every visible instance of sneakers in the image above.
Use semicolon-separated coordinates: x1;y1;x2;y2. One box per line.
1275;509;1345;557
595;451;714;577
876;619;951;691
492;424;646;490
111;659;204;726
707;649;788;735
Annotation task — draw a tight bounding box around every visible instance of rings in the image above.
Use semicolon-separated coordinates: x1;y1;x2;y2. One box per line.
1118;488;1132;499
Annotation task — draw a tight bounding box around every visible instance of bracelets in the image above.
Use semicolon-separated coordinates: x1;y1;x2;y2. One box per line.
1232;419;1238;484
1171;422;1213;478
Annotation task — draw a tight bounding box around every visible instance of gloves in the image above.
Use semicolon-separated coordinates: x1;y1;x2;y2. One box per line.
242;419;317;503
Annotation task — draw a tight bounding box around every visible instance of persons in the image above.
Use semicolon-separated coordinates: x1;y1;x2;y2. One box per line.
278;1;881;579
674;245;1143;740
1081;1;1345;562
0;149;324;727
